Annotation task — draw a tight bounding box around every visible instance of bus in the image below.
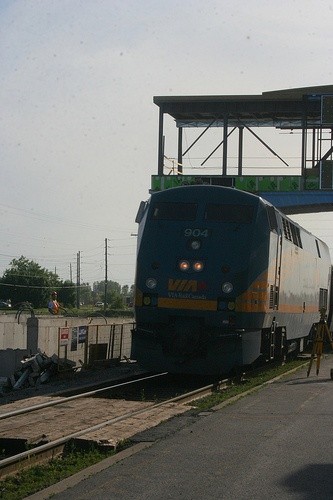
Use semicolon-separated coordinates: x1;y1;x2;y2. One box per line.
129;184;333;371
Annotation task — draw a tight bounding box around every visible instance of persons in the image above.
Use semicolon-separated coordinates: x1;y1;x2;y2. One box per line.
48;291;60;314
